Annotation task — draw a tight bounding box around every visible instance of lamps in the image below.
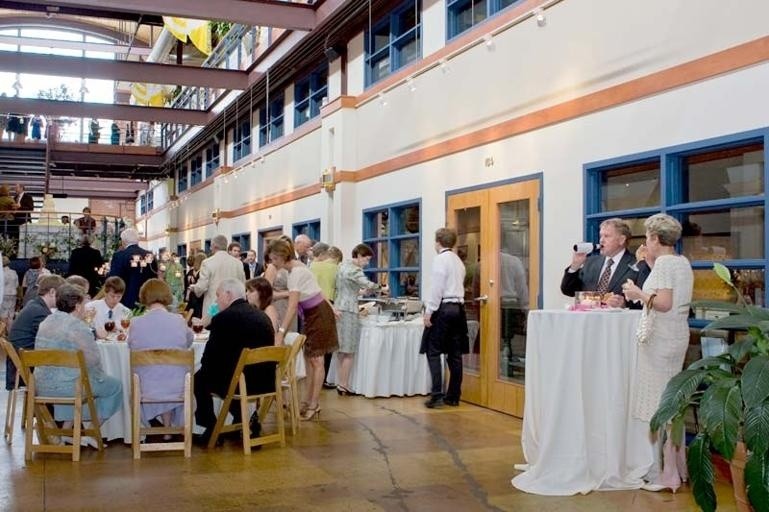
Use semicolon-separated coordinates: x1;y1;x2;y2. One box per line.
130;253;182;280
210;208;219;224
164;224;170;236
319;166;336;192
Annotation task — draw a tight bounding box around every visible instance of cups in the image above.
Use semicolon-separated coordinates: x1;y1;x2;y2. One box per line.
574;291;602;306
192;319;203;333
121;319;130;334
84;303;95;321
86;322;97;340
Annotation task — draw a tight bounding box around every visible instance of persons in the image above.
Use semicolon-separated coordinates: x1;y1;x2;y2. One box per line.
128;278;195;444
1;182;34;258
182;231;382;397
620;212;690;493
193;276;277;452
63;207;96;246
1;228;184;356
560;219;651;309
88;117;101;144
0;113;61;145
456;245;474;289
6;275;133;449
110;121;156;148
264;238;339;422
419;228;471;408
473;225;530;377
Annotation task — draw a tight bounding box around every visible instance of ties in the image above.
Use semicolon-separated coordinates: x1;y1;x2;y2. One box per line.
109;311;112;318
596;259;613;291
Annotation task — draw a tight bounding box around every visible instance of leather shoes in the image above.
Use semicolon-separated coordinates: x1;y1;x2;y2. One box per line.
425;398;458;406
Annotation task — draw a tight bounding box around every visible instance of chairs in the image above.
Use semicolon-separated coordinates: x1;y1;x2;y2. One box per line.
205;342;292;456
244;334;307;437
129;349;196;460
1;338;63;446
175;309;193;322
19;347;105;461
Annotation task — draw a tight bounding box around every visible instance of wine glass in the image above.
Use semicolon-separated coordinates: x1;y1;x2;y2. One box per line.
628;244;649;272
104;319;116;338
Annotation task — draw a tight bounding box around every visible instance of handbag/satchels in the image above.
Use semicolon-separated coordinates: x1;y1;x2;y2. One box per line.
636;295;657;345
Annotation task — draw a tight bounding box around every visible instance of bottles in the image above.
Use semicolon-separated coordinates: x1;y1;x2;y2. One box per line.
573;242;603;254
730;269;764;306
380;271;389;297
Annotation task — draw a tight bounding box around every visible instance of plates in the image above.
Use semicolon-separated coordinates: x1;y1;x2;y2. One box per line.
369;315;389;322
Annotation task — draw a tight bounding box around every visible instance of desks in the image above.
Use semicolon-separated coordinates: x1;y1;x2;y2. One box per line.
59;338;260;453
511;309;663;498
326;309;445;398
19;224;79;259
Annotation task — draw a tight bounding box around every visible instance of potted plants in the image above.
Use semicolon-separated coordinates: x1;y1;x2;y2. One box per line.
650;262;769;512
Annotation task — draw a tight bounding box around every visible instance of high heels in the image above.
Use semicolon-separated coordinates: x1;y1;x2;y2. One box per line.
641;481;680;494
337;385;356;396
300;402;321;421
61;435;108;449
